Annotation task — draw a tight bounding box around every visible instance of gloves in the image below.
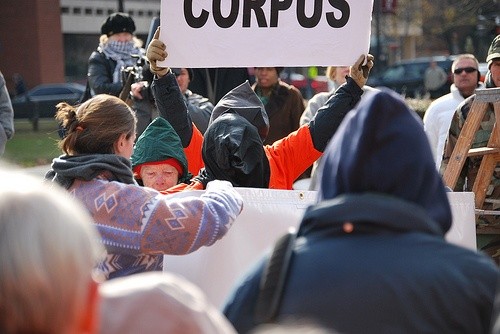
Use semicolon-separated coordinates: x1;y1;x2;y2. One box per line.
147;26;170;76
349;54;374;88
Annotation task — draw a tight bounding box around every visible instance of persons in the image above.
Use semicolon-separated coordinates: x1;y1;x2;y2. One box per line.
300;66;372;190
0;171;102;334
441;36;499;265
87;13;146;103
147;27;374;195
131;117;191;192
46;95;242;279
130;69;213;142
0;71;14;157
223;92;500;334
424;53;483;169
251;67;304;144
160;114;270;193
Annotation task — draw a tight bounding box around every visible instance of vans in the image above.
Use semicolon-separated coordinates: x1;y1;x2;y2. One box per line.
364;55;462;98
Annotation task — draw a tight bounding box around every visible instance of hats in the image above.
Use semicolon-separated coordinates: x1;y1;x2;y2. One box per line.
485;35;500;63
133;157;183;179
100;12;136;37
254;67;284;77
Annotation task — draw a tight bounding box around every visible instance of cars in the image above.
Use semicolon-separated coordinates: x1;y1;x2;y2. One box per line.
10;83;96;118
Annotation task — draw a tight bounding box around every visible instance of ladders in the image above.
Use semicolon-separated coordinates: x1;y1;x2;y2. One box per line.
439;87;500;265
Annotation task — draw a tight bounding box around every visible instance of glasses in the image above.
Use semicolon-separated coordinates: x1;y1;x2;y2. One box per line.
451;67;479;77
